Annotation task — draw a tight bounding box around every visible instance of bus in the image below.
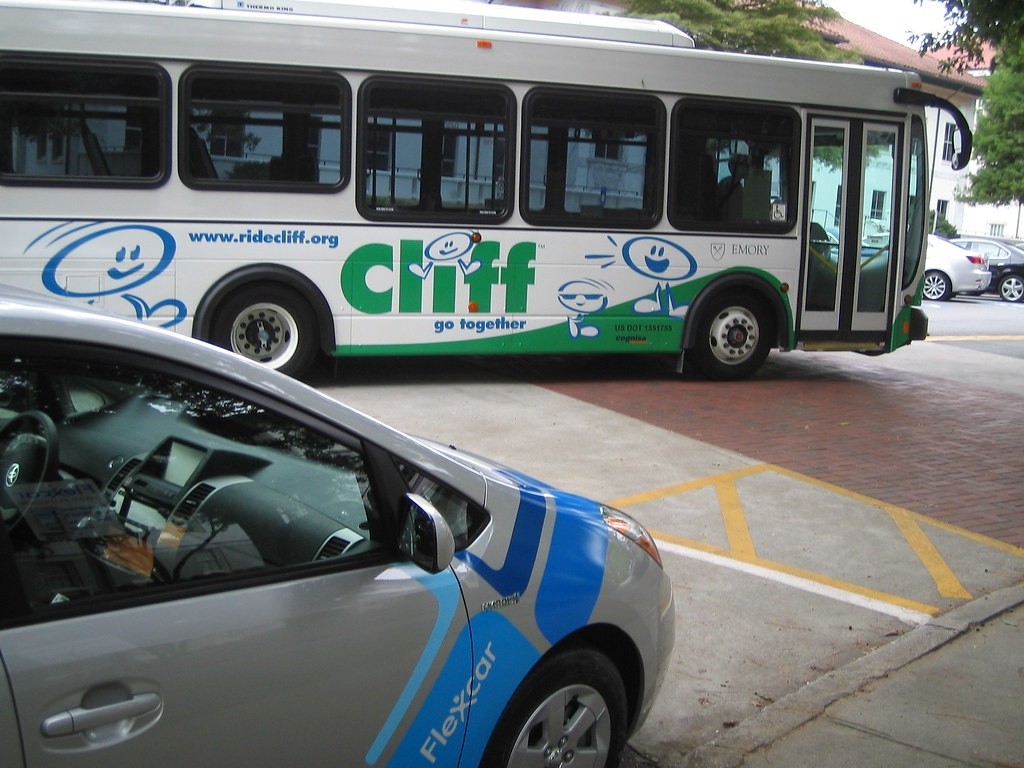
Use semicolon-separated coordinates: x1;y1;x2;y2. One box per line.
1;2;976;380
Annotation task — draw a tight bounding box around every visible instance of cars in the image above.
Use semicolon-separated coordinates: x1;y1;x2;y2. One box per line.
0;282;676;767
823;227;882;270
827;233;992;301
949;237;1024;304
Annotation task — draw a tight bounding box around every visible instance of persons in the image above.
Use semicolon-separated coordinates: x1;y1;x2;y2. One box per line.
715;152;750;221
